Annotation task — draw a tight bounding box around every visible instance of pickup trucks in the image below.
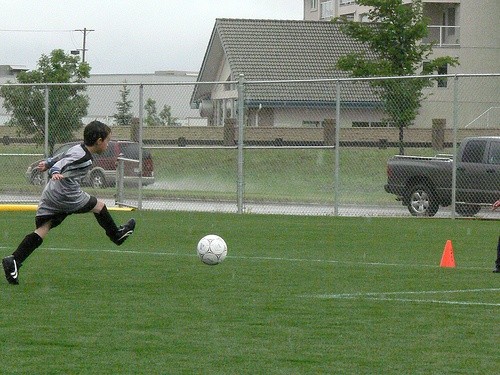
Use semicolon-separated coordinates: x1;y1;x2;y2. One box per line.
382;135;499;217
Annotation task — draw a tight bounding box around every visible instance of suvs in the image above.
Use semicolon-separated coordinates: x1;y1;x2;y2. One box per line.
23;139;157;189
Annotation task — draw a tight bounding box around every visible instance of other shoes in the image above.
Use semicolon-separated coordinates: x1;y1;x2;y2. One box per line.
493;267;500;272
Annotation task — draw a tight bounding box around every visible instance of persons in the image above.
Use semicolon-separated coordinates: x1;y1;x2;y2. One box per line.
2;121;136;285
492;198;500;273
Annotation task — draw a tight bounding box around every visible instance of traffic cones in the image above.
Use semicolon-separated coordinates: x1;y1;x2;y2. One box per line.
439;240;457;269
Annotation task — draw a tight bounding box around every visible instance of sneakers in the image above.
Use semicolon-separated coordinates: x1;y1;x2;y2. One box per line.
2;254;23;286
109;218;136;246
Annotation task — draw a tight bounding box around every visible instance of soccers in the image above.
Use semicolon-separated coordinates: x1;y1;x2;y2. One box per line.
196;233;228;266
